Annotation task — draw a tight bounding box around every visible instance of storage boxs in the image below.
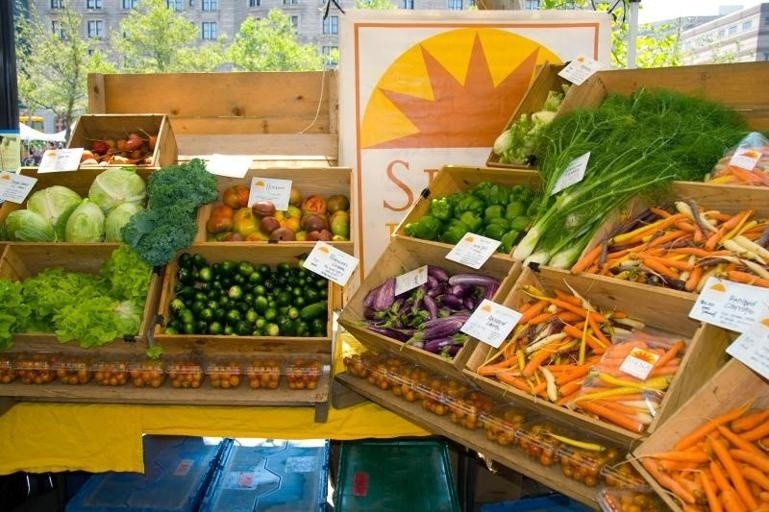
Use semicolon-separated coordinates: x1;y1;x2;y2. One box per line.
540;182;768;296
65;114;178;172
192;166;355;244
623;359;767;510
337;238;521;389
1;243;162;354
153;247;334;355
487;64;578;170
392;165;546;264
465;265;732;450
1;166;160;245
540;61;766;188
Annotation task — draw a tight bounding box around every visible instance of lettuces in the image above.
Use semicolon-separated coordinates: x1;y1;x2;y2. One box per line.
0;246;154;349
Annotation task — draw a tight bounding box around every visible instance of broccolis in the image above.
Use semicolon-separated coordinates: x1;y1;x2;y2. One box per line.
123;160;218;269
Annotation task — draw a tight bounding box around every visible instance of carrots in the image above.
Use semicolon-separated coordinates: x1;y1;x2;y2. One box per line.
644;399;769;512
476;276;685;433
569;196;768;296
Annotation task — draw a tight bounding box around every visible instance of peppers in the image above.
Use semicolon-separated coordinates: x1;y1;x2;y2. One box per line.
404;180;540;254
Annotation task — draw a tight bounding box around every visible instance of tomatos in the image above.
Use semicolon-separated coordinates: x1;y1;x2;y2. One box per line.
0;356;321;390
343;351;668;511
204;186;351;244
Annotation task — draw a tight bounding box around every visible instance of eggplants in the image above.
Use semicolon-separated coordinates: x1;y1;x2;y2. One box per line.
363;264;502;363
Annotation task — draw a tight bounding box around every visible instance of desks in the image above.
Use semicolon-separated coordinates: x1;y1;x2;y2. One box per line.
4;402;433;479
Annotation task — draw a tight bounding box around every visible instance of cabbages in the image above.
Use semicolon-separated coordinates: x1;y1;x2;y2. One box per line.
3;169;146;245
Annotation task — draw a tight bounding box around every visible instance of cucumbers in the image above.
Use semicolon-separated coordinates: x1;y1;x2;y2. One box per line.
162;253;328;337
